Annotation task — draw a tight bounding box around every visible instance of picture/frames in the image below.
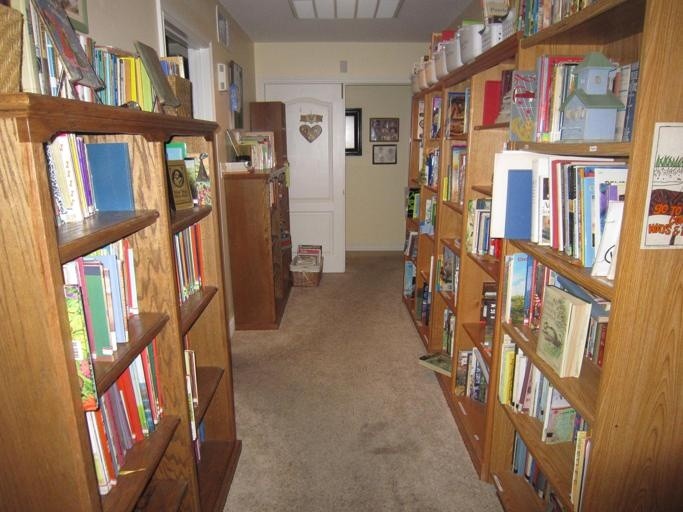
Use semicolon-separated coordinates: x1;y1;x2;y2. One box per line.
368;117;399;166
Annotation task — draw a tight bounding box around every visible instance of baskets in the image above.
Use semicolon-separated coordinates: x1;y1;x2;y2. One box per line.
289;256;324;287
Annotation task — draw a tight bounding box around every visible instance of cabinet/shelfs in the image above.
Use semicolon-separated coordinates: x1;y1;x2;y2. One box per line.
0;94;244;511
223;101;293;332
402;2;683;512
